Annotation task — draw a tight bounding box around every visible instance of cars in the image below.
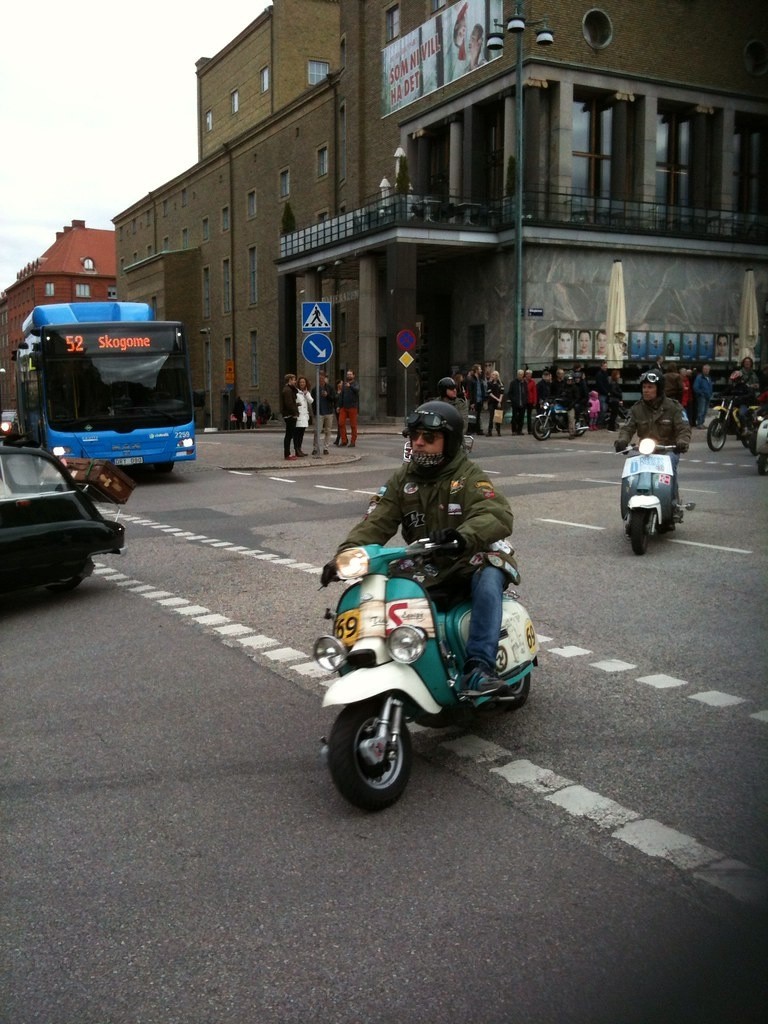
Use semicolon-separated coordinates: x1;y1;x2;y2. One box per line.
0;447;125;597
0;411;17;435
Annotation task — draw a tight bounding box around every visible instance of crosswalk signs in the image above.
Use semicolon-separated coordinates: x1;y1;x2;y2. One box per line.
301;301;333;335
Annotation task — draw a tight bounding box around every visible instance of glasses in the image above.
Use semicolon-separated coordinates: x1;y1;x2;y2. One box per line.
640;374;659;383
407;411;448;429
410;431;443;443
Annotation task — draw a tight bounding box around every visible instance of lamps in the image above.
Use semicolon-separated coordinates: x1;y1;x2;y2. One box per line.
379;179;391;188
394;147;407;157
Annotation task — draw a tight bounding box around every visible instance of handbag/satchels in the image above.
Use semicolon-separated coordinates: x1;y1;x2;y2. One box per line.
493;408;503;423
466;409;476;423
308;404;314;418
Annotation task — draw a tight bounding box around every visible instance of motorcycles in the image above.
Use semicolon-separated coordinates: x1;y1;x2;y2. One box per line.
705;383;765;454
531;396;591;443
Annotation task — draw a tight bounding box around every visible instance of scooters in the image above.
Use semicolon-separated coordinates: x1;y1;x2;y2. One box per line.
749;413;768;476
305;533;538;809
616;438;697;555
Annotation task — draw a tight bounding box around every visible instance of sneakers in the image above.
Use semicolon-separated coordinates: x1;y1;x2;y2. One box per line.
458;664;508;695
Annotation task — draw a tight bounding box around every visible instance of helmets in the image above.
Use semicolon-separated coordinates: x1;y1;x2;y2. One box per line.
574;373;581;379
438;377;457;397
563;374;572;383
408;401;464;461
640;369;666;396
730;371;742;381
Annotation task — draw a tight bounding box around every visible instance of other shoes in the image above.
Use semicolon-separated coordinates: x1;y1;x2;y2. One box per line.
477;431;484;435
287;448;330;460
741;427;748;436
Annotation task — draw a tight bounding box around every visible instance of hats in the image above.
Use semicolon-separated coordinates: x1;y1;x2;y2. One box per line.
590;391;598;397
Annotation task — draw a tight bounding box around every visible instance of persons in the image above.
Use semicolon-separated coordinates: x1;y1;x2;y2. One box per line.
334;370;360;447
257;399;270;424
279;374;302;459
730;357;768;438
717;334;740;357
559;329;574;355
432;377;468;435
233;396;254;430
596;331;607;355
667;339;674;356
507;362;624;439
320;401;520;696
454;364;504;437
653;355;713;428
578;331;591;356
293;377;314;456
312;371;336;455
463;27;483;73
614;369;692;521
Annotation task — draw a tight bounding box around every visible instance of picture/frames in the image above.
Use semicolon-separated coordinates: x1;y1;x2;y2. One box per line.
556;328;761;362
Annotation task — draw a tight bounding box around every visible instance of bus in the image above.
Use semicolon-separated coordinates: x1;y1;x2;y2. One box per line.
8;301;197;471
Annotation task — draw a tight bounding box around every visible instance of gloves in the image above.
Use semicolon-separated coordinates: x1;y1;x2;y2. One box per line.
321;559;340;586
614;440;628;455
731;391;738;395
674;441;689;455
429;528;465;555
714;393;721;398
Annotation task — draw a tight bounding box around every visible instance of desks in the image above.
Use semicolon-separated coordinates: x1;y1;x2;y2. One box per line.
456;203;481;225
416;200;441;222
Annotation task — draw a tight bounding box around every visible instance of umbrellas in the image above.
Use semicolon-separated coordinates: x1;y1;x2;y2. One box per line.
605;260;628;368
737;269;759;370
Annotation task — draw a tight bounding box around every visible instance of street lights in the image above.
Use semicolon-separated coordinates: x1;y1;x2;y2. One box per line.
484;0;555;423
201;326;215;427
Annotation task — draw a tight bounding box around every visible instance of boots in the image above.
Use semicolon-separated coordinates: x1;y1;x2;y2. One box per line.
593;423;598;430
589;424;593;430
